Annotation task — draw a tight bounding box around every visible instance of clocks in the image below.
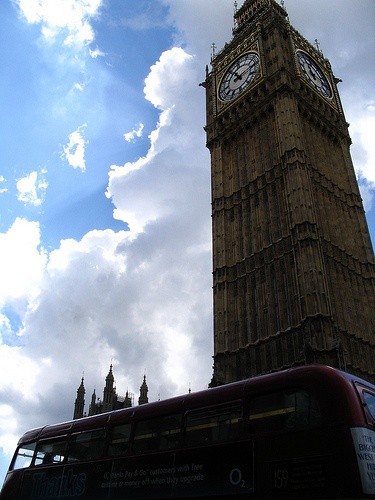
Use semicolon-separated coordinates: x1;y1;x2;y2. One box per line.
217;51;261;103
295;49;334;100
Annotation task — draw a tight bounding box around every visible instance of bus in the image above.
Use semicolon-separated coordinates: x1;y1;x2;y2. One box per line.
0;363;375;500
